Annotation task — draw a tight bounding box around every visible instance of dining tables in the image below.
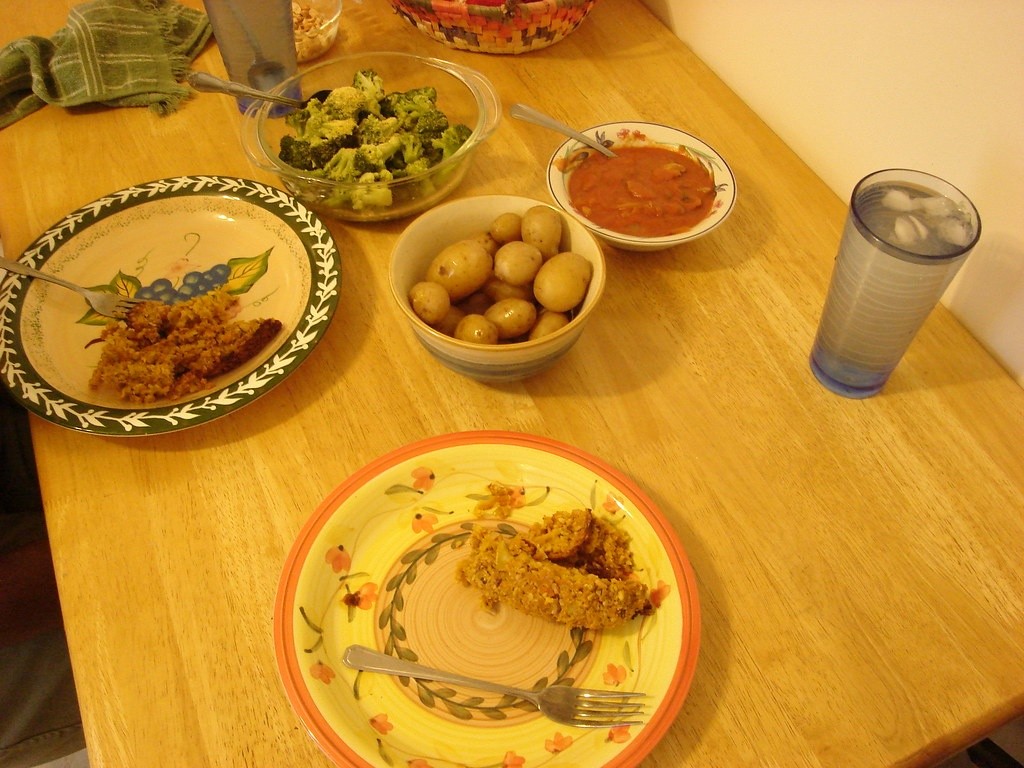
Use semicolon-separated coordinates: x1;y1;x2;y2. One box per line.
0;1;1024;768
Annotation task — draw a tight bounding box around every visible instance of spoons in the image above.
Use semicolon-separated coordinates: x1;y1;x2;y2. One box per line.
187;70;335;111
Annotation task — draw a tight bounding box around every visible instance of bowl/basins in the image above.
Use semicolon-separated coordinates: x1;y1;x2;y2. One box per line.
547;120;737;252
238;50;502;223
388;192;607;382
294;0;343;64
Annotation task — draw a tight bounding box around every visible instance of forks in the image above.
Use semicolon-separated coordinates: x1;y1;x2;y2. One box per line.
0;256;156;319
342;645;647;726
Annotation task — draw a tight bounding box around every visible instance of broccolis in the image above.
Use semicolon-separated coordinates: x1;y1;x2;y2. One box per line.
278;68;473;215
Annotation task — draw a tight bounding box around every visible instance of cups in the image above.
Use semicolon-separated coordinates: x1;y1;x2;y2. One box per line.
809;168;982;398
203;0;303;118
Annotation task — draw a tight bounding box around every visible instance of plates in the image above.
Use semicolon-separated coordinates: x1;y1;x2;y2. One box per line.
273;429;700;768
0;174;343;438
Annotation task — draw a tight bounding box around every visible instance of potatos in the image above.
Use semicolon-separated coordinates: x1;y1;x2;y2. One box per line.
409;205;592;344
